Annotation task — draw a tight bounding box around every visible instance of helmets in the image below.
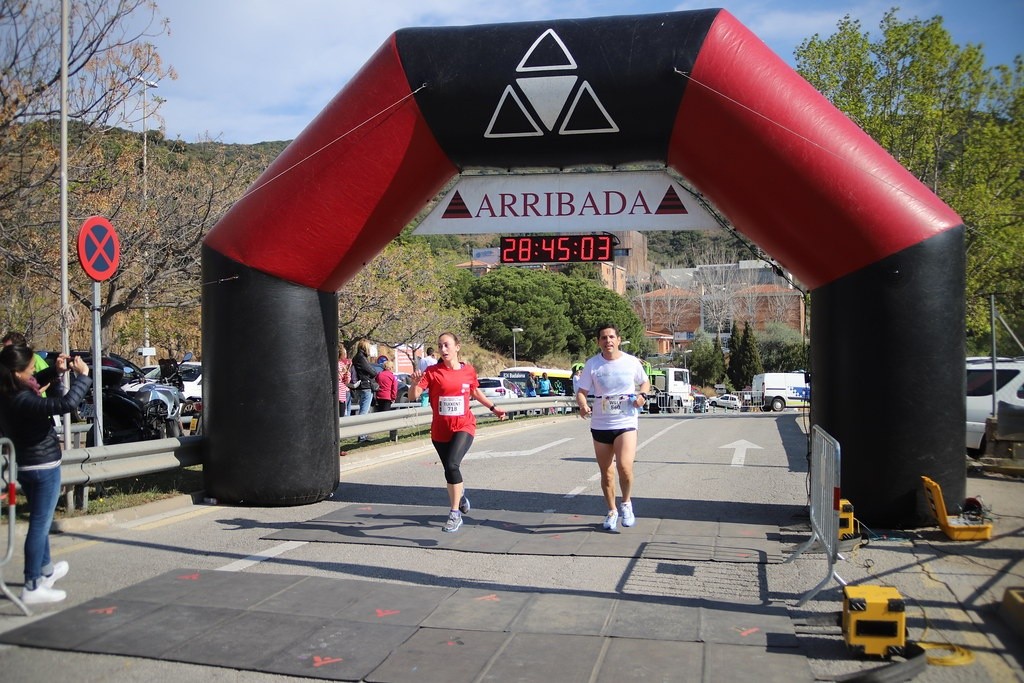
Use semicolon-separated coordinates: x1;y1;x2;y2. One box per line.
377;355;388;366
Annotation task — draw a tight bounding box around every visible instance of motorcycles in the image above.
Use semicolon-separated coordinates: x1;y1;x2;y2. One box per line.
86;351;192;451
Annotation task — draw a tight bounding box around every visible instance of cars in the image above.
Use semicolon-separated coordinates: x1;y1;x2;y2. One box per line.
476;377;520;399
964;356;1024;460
369;362;415;409
692;395;709;412
709;394;741;410
33;348;203;429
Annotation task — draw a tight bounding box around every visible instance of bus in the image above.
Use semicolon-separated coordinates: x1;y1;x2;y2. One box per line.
498;367;574;397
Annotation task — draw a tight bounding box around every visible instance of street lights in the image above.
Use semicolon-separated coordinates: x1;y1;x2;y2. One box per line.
512;329;524;366
132;75;158;365
683;350;692;369
620;341;630;351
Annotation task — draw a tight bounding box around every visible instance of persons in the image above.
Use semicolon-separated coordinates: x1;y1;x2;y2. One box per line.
417;348;438;408
352;340;377;415
0;344;93;603
375;360;397;411
408;332;507;533
575;324;651;527
573;370;582;395
525;372;539;397
538;372;552;396
338;343;351;417
1;331;51;418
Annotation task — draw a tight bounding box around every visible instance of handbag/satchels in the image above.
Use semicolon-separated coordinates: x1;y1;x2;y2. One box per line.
370;379;379;391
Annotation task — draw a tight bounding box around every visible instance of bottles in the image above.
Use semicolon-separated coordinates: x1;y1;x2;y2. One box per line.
630;394;644;412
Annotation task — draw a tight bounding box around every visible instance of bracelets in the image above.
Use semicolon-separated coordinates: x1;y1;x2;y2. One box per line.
490;404;497;411
640;393;647;399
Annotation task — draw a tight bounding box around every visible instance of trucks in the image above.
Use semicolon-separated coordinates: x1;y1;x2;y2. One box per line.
751;372;810;412
641;360;691;413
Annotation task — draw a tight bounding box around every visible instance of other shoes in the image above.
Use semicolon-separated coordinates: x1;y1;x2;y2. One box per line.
359;437;376;443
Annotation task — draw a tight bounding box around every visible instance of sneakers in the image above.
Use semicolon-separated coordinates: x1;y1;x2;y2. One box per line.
40;560;69;588
442;511;463;533
22;583;67;603
620;501;636;527
603;509;618;530
458;489;470;514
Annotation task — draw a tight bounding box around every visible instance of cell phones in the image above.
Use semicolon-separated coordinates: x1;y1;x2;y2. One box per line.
66;357;76;368
